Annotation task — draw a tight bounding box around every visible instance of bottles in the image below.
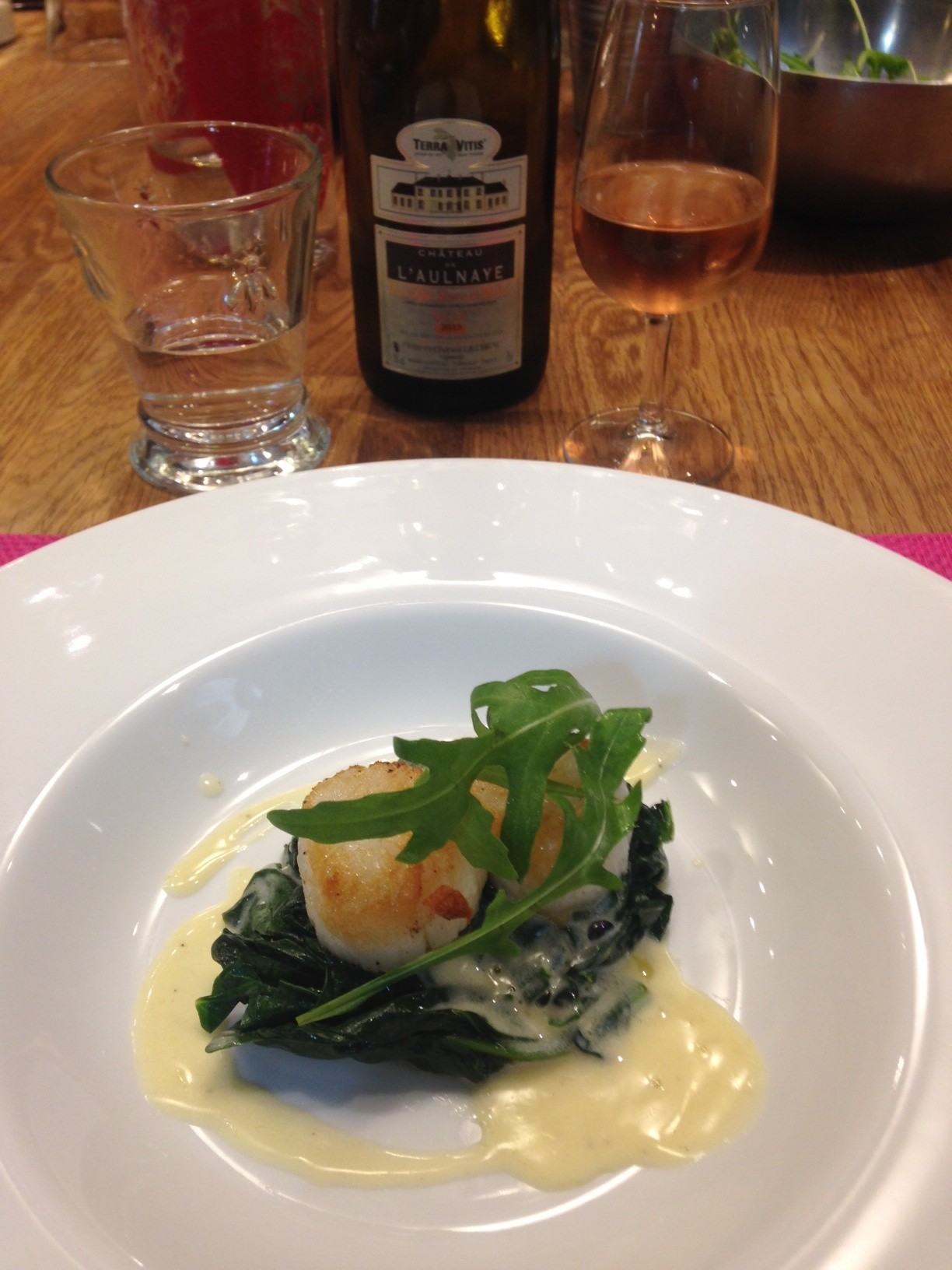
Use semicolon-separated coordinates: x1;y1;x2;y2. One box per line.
329;0;563;417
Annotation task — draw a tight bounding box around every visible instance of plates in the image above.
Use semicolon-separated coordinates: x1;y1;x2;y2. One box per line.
0;457;952;1269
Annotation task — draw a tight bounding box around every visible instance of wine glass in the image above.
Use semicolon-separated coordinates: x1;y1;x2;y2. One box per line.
562;0;783;480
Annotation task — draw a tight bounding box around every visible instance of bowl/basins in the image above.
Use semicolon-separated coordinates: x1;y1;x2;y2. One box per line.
672;0;952;193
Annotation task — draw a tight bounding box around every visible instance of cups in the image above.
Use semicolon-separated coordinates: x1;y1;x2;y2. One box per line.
44;120;331;496
121;0;334;276
44;0;128;67
567;0;672;136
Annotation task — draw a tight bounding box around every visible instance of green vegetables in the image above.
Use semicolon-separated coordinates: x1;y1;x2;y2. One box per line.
706;1;940;85
194;663;674;1077
